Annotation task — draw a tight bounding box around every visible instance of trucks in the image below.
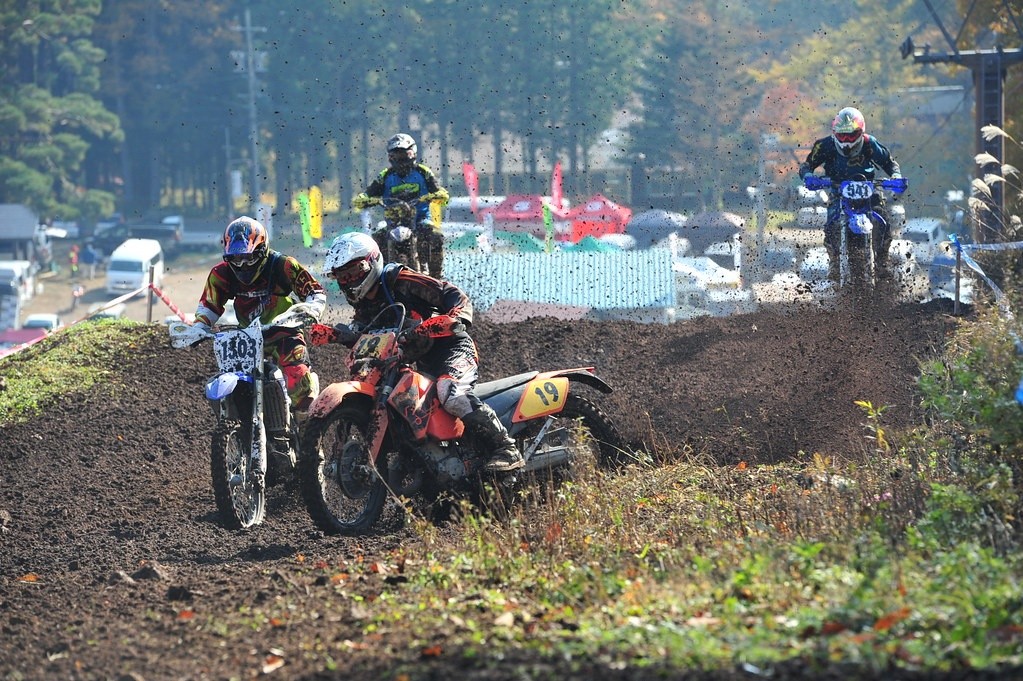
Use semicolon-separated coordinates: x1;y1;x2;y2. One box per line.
156;214;224;256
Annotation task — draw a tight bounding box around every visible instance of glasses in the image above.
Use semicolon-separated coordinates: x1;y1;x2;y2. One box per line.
333;259;372;285
833;129;862;143
389;150;416;162
224;250;262;265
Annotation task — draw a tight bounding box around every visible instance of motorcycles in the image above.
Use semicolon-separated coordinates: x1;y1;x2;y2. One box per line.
294;301;628;537
167;300;325;532
352;191;451;280
804;174;911;311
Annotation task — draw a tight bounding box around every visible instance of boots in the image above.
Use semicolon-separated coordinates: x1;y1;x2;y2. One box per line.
824;240;841;283
873;238;892;277
461;405;526;473
295;410;325;486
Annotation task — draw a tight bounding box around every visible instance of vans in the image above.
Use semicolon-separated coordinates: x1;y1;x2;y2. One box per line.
106;237;164;297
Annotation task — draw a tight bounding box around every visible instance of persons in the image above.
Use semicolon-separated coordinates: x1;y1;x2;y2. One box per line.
65;244;80;277
304;232;527;471
82;242;97;277
193;215;326;411
354;133;450;279
798;106;902;293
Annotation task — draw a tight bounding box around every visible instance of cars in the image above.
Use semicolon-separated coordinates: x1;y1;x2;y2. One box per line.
93;219;126;241
902;217;946;264
51;220;81;240
86;300;128;320
21;312;61;334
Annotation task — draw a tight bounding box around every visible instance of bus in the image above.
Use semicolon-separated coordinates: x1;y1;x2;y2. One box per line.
443;194;573;243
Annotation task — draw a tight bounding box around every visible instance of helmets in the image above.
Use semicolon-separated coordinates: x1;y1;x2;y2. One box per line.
322;233;382;303
831;108;865;158
222;216;269;286
386;132;418;177
